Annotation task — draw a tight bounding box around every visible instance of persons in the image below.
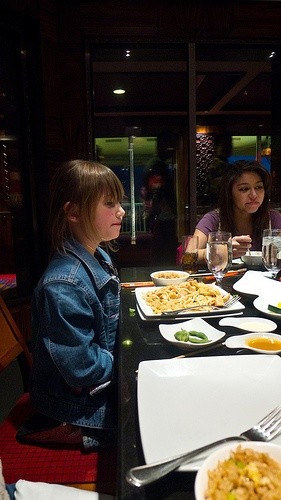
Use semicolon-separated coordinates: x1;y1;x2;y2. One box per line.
140;158;176;268
184;159;281;264
30;160;126;451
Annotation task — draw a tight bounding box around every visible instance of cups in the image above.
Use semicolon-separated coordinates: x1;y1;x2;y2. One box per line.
182;235;199;274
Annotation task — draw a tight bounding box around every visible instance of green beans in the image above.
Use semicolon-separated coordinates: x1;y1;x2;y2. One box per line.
174;330;212;343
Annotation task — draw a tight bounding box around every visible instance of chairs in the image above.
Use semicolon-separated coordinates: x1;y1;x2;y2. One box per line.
0;293;116;496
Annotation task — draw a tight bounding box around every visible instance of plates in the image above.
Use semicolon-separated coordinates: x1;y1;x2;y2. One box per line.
231;259;244;269
158;317;226;349
141;354;281;474
134;284;246;320
225;332;281;354
253;284;281;320
233;270;280;295
219;316;277;332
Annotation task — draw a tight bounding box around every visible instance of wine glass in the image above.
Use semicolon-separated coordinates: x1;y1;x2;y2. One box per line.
206;232;232;285
262;229;281;279
206;241;233;293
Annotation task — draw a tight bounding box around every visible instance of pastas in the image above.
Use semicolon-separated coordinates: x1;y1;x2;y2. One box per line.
145;287;215;314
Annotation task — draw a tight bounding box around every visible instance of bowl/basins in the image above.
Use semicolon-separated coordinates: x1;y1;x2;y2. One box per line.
150;270;189;285
242;249;264;265
195;440;281;500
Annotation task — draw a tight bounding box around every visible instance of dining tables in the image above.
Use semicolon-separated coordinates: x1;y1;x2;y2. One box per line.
112;258;281;500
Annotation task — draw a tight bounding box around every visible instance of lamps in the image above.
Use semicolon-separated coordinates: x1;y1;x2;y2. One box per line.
261;135;272;158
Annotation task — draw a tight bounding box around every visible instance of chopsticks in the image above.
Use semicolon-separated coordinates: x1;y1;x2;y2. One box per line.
136;342;226;376
206;272;238;279
124;284;154;287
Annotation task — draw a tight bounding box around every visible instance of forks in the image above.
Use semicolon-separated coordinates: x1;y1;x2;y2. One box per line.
160;296;240;315
124;404;281;487
